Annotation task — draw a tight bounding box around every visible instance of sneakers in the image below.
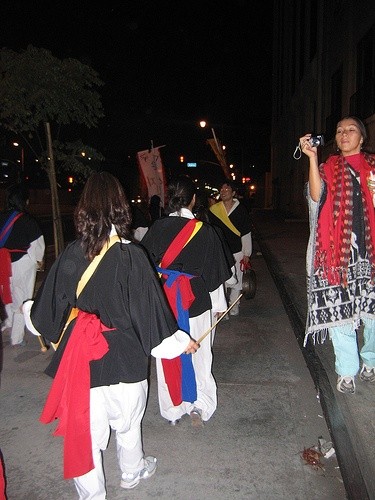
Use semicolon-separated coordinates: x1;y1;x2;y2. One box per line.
120;455;158;490
358;363;375;382
336;374;357;395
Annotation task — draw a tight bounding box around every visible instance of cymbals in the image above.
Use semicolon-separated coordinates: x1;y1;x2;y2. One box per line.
238;259;256;300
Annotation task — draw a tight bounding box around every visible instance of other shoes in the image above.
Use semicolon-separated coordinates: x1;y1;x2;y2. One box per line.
165;418;180;425
190;407;206;429
11;340;28;349
229;305;240;316
219;313;229;322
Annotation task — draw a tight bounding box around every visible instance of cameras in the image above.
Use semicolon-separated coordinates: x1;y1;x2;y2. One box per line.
309;134;325;147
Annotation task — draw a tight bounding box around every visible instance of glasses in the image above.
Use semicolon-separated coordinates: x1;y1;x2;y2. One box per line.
220;188;233;192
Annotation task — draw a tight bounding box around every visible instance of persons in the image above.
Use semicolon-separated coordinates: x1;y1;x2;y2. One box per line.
139;176;229;426
1;182;45;349
299;115;375;394
20;172;200;500
148;180;252;321
